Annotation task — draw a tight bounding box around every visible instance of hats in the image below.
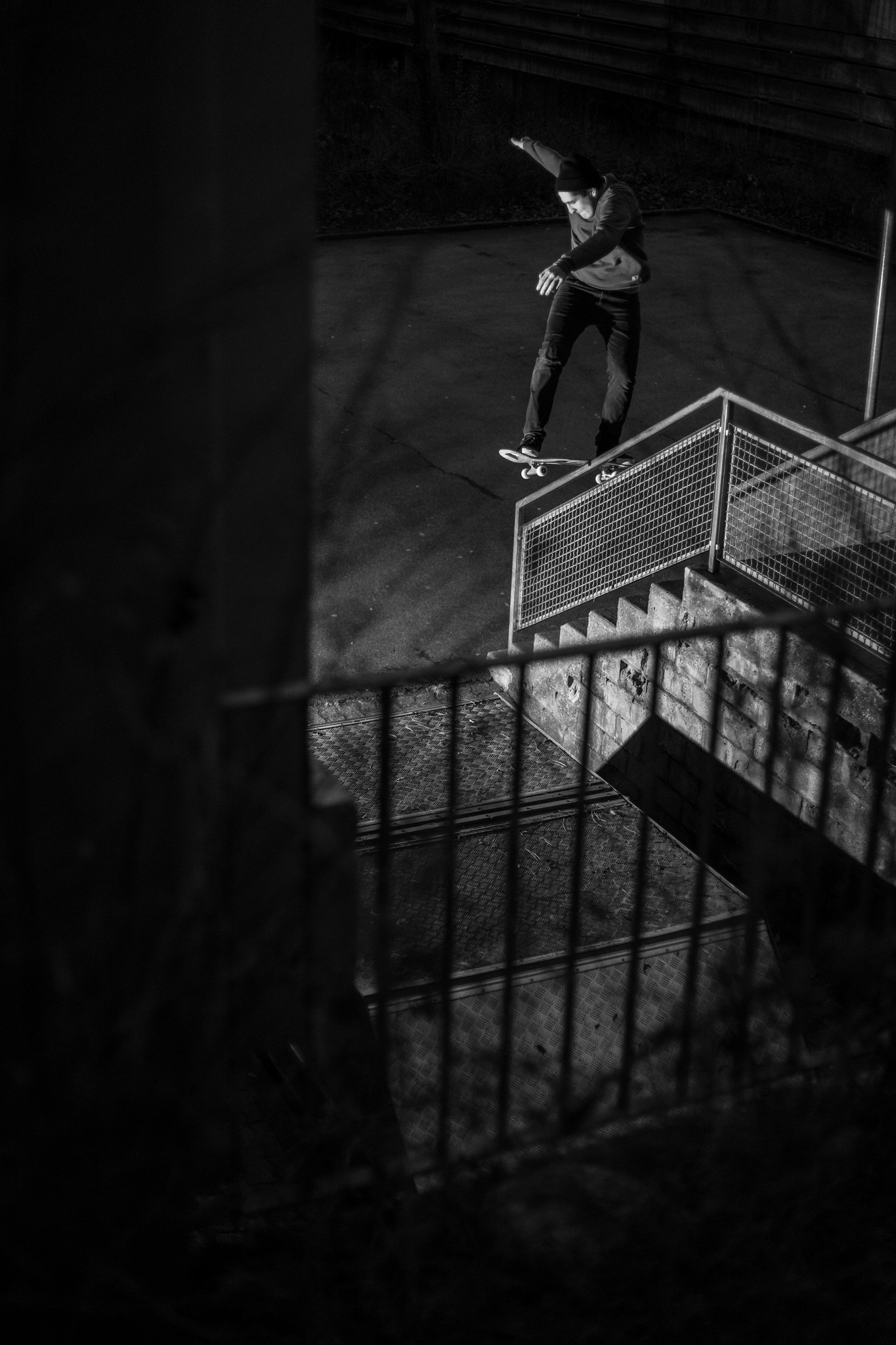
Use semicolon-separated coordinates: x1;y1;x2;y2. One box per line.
554;155;603;192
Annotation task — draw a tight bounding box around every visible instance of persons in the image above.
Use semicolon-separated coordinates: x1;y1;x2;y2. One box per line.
508;133;648;463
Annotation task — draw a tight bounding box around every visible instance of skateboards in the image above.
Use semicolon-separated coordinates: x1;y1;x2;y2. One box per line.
499;449;631;485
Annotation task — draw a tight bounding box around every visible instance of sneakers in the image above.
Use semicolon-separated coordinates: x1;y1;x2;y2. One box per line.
516;434;542;457
595;447;633;465
